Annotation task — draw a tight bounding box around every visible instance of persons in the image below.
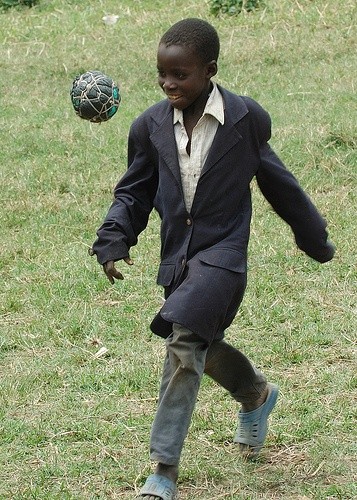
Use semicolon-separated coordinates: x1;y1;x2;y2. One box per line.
88;19;336;500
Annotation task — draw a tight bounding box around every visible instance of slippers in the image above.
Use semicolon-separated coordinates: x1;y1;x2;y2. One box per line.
135;473;178;500
234;384;278;458
